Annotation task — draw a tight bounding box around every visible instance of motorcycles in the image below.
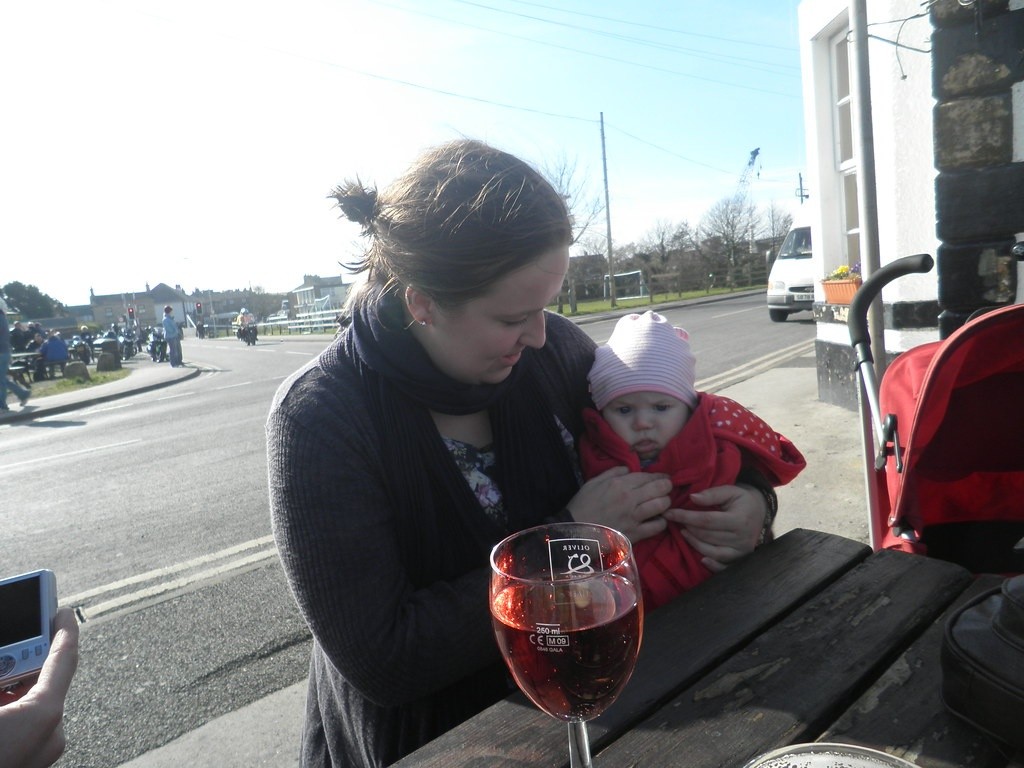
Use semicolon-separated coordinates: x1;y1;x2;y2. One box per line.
71;328;167;364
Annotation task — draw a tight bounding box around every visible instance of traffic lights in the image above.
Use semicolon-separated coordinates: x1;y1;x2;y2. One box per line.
196;302;202;313
128;308;134;319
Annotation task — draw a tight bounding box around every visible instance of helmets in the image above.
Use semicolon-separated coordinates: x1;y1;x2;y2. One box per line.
241;308;249;314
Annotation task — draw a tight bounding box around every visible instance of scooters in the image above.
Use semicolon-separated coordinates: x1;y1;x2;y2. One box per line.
237;324;259;345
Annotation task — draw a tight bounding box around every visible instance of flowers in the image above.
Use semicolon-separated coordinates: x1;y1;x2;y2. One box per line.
823;263;861;282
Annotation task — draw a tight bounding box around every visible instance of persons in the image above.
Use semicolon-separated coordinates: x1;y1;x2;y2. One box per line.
238;308;255;337
80;326;94;364
10;320;68;381
577;310;741;608
0;308;31;414
162;305;185;367
264;139;778;768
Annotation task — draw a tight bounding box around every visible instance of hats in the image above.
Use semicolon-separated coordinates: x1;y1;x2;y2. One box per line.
164;306;173;314
588;310;698;412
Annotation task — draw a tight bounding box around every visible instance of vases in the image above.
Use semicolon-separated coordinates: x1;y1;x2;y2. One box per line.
822;279;863;304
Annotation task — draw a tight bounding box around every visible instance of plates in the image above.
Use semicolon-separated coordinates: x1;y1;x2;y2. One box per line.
742;743;921;768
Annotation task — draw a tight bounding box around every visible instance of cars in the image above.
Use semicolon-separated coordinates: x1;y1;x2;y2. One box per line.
767;199;814;320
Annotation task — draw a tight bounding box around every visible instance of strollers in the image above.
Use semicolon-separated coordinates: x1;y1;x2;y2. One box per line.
848;243;1024;574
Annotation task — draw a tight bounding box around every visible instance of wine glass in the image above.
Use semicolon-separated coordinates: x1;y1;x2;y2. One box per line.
487;524;645;768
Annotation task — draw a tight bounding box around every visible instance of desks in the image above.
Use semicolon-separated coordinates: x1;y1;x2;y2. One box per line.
387;528;1024;768
9;353;42;385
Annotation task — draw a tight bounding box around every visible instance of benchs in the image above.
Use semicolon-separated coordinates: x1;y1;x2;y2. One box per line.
8;366;31;390
41;359;82;379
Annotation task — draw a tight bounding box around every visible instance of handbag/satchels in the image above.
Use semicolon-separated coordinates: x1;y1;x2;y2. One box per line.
937;572;1024;753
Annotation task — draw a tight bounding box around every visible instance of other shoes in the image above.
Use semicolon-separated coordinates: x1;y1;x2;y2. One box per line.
172;362;184;368
0;406;9;412
20;390;32;406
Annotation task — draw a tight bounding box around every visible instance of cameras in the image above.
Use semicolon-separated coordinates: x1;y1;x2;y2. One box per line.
0;569;58;690
